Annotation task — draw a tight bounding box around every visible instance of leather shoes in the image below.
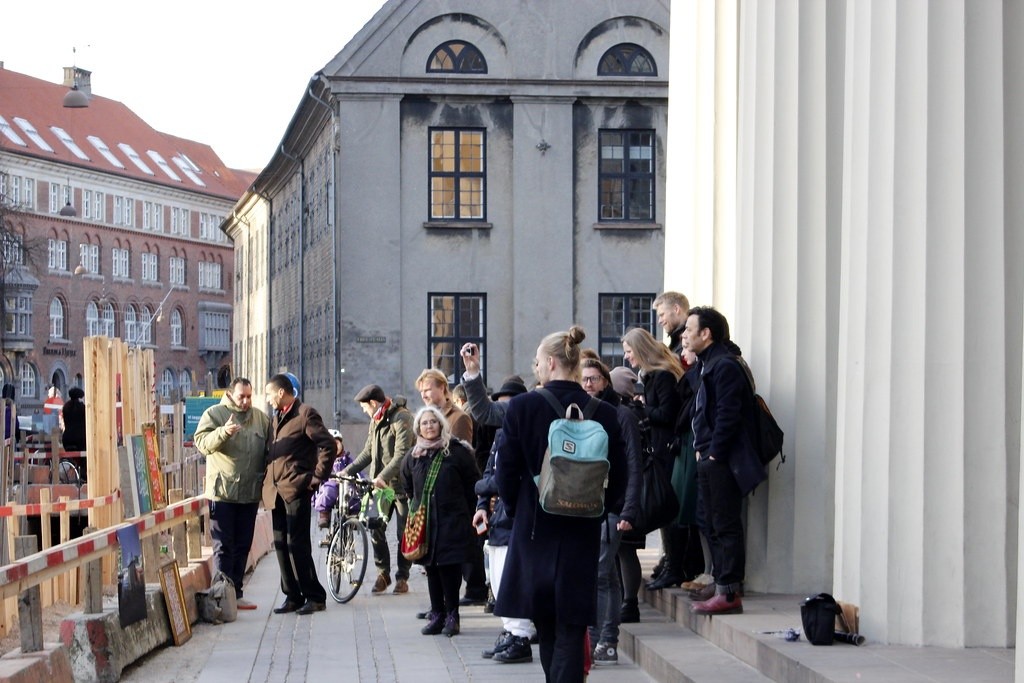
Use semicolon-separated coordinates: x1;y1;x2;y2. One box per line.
274;600;304;613
296;600;326;614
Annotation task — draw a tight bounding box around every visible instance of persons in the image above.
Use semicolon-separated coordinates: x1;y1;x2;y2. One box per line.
0;384;21;443
402;408;481;636
449;328;649;683
61;387;86;465
622;290;786;615
336;383;416;594
264;375;336;615
415;367;490;605
313;430;366;526
192;377;272;610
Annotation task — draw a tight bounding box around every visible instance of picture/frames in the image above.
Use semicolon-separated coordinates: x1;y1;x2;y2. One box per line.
158;559;192;646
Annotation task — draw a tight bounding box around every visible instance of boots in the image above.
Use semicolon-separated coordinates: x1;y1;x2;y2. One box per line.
621;598;640;623
645;561;668;587
319;511;329;527
648;560;686;590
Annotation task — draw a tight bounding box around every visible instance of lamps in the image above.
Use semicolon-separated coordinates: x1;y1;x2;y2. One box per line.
59;177;76;216
98;296;108;305
156;310;166;322
74;264;86;275
62;85;89;108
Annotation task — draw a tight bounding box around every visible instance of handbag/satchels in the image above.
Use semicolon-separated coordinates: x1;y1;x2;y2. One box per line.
640;454;680;535
401;493;429;560
195;571;238;624
800;593;842;646
736;356;784;461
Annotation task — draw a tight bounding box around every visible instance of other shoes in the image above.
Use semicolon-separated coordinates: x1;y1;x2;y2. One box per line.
681;573;743;615
417;583;496;636
237;598;257;609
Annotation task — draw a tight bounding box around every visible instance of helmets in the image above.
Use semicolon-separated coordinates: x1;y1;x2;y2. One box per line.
328;429;343;441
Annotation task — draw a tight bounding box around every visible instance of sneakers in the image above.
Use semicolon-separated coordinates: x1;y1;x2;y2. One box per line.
392;579;408;594
492;636;532;662
482;632;520;658
372;574;391;592
593;642;618;665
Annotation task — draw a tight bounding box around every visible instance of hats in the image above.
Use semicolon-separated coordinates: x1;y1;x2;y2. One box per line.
609;366;638;398
491;375;527;401
354;385;384;402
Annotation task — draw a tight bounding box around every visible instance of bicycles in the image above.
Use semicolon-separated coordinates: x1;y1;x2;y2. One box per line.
320;474;388;603
48;445;82;485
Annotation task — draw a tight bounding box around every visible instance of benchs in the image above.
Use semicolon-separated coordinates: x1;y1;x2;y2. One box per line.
14;462;50;483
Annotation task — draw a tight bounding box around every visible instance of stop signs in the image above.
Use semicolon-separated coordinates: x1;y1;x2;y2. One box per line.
44;397;64;416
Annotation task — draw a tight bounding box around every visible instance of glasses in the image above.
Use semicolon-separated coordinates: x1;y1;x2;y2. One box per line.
582;375;604;385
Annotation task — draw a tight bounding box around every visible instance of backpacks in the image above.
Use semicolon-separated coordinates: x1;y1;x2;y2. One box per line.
528;387;611;517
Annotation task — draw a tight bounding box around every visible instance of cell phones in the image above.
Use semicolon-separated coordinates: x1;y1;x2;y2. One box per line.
476;519;487;535
